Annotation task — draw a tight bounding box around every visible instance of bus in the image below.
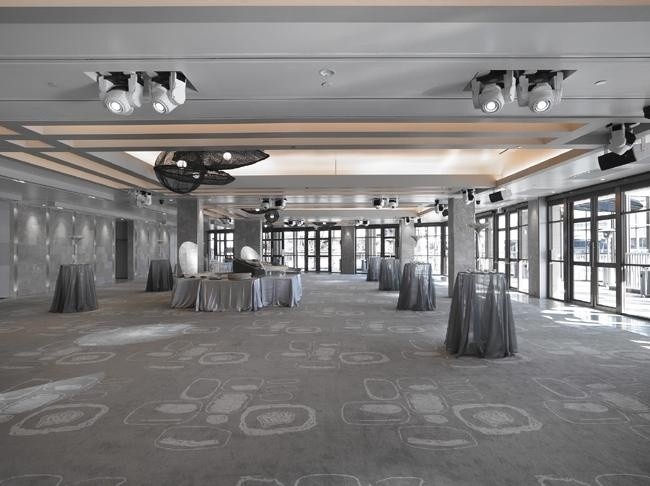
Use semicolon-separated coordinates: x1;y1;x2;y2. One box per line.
573;239;608;254
630;237;647;253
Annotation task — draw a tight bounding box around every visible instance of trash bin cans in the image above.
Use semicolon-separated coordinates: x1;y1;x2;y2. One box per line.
361;260;367;272
523;263;529;279
640;270;650;298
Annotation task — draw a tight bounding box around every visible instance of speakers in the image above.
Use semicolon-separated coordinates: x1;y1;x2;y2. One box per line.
489;185;513;203
597;136;650;172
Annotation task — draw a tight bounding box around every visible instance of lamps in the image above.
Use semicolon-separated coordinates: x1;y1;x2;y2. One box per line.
220;152;237;163
147;71;189;116
174;154;190;173
127;188;511;230
462;69;517;114
97;73;145;115
517;70;576;113
602;123;638;155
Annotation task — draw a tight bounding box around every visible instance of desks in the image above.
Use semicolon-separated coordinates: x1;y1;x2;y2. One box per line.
169;268;302;312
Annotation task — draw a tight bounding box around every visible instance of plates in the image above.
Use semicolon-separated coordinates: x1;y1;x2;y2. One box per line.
209;272;252;280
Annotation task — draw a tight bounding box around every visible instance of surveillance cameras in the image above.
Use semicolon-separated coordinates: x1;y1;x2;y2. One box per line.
159;200;164;204
476;200;480;204
